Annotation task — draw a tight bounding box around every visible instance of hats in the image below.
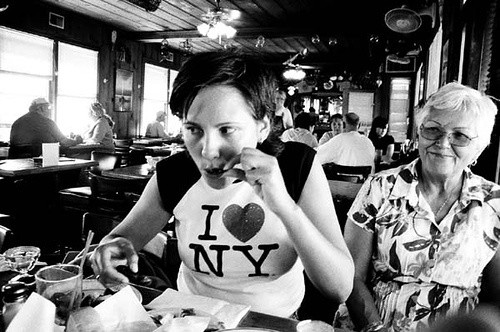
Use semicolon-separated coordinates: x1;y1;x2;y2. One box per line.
31;98;48;105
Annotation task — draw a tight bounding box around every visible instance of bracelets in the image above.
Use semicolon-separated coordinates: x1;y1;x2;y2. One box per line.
360;318;386;332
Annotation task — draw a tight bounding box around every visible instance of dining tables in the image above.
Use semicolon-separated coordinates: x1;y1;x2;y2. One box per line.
0;250;352;332
68;144;102;149
101;163;154;180
0;158;99;178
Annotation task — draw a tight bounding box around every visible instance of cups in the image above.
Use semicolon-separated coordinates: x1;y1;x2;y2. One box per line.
296;319;334;332
34;264;83;325
376;150;383;162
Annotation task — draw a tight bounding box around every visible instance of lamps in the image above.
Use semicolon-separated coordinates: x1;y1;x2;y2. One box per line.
197;0;242;45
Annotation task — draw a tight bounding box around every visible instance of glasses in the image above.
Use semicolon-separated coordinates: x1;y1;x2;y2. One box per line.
419;120;478;147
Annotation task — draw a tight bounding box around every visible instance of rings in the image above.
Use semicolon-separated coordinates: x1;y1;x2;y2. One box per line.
96;274;101;280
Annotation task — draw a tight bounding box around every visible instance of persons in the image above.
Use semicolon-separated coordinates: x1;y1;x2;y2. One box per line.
9;98;83;158
313;113;375;181
145;111;171;138
281;112;319;148
345;82;500;332
368;115;395;164
83;102;115;145
273;90;294;129
318;113;344;147
90;49;355;319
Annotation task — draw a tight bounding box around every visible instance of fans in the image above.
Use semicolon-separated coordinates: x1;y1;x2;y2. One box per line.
383;2;436;33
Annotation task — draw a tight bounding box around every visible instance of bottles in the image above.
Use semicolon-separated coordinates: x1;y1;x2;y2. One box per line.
2;283;27;328
399;144;405;159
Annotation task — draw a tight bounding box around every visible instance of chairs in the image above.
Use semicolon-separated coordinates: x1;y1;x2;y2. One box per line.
0;144;500;325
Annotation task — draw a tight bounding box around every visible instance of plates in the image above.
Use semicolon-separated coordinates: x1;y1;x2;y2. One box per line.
42;279;142;308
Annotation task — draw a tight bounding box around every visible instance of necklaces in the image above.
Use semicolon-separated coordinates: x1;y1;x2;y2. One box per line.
432;188;457;218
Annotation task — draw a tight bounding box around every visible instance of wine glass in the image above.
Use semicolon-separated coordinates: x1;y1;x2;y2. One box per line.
3;246;40;285
